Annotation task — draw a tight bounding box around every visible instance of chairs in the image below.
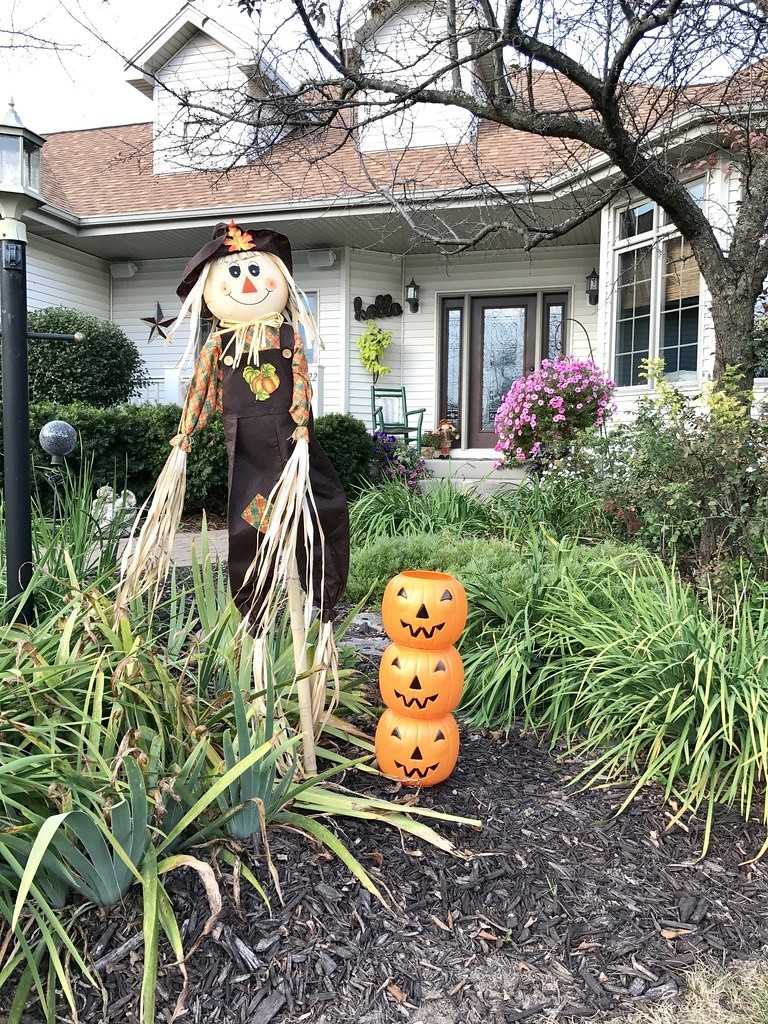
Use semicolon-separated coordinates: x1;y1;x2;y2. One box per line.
371;385;426;458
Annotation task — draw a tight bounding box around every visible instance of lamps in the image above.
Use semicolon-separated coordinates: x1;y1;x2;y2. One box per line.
585;267;599;306
405;277;420;313
307;251;336;268
0;97;48;219
109;263;138;278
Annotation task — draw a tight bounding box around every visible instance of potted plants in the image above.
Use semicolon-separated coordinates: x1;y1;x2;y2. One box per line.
420;431;441;460
